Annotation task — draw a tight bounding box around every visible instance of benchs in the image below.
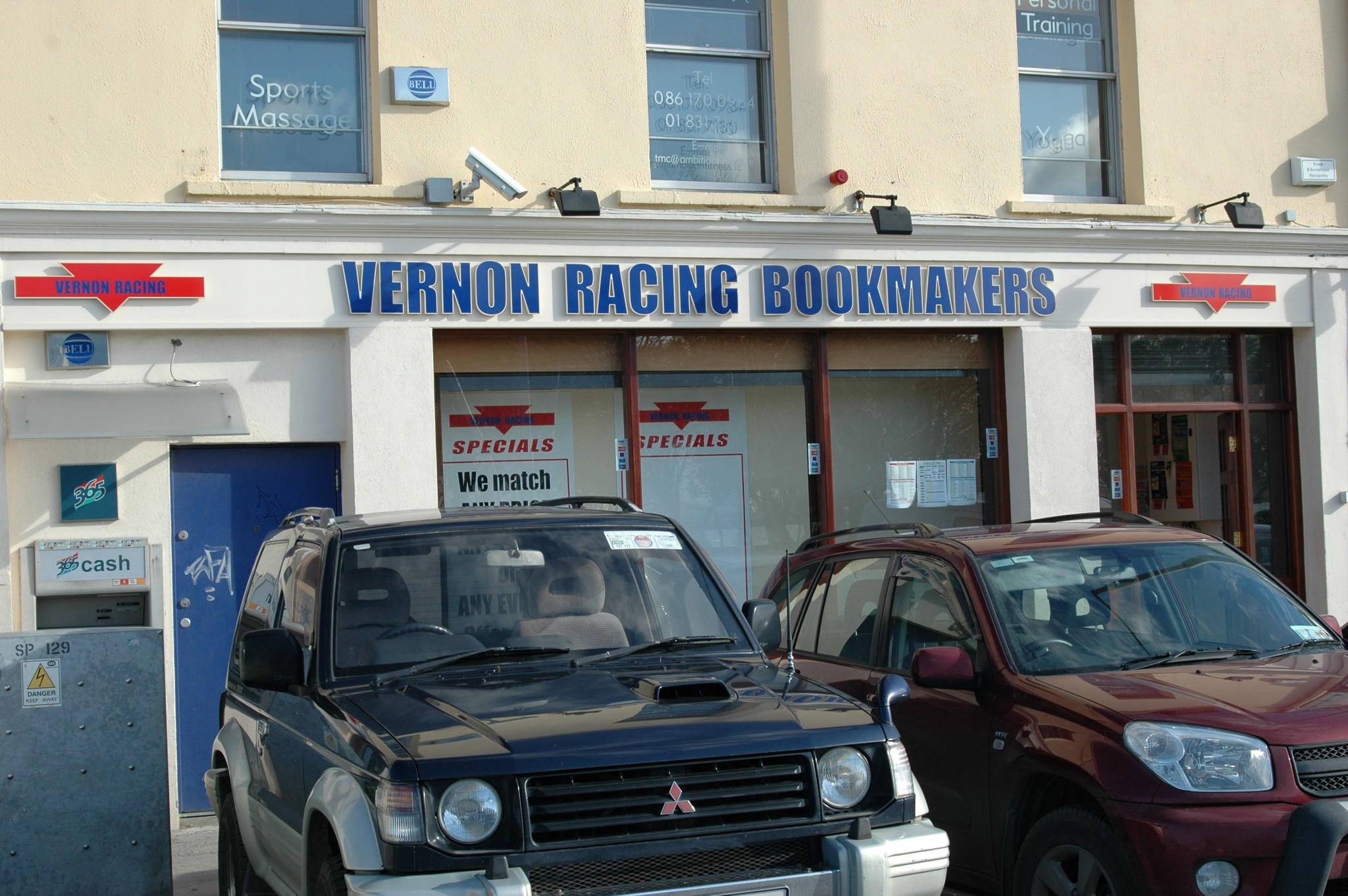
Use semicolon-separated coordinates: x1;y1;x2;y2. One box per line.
811;579;885;654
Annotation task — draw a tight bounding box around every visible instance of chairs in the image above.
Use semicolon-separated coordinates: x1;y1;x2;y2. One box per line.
335;567;430;666
1058;574;1129;654
914;588;960;648
512;556;629;649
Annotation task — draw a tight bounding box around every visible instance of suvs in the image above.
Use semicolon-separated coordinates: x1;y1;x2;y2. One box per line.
754;507;1348;896
205;495;954;896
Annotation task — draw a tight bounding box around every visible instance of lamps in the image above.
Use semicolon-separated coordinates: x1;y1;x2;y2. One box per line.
548;177;601;216
1194;191;1265;229
853;191;913;234
